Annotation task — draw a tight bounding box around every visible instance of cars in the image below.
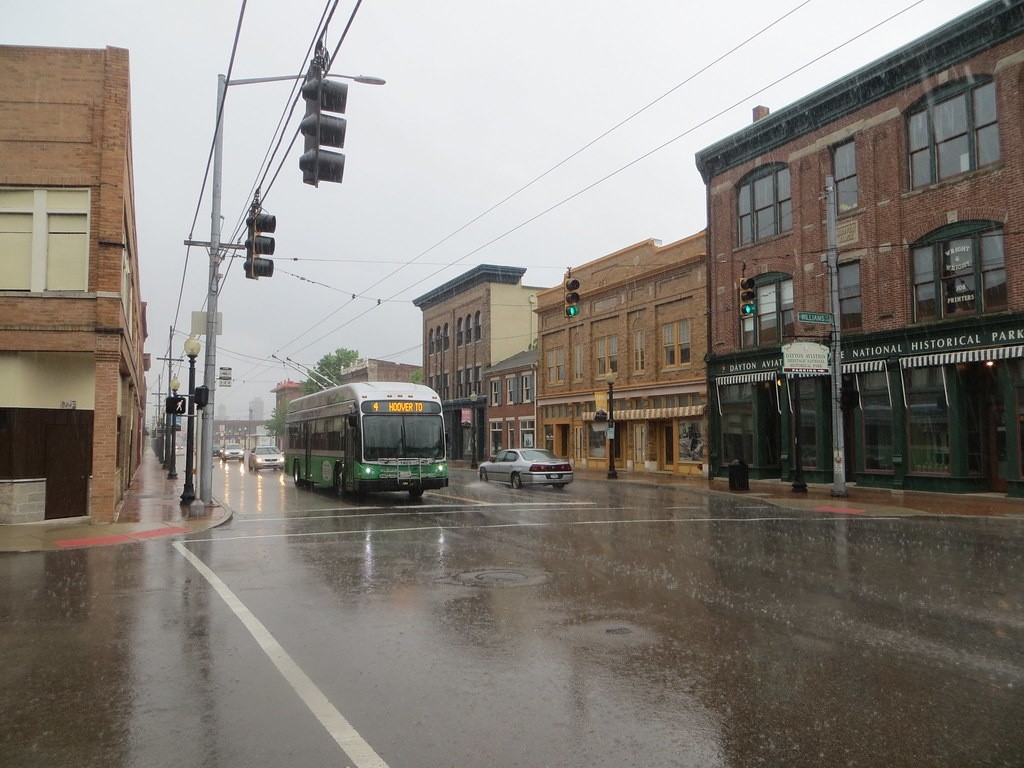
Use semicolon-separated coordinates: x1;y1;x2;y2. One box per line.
478;448;573;489
175;444;185;455
248;446;285;471
212;443;245;463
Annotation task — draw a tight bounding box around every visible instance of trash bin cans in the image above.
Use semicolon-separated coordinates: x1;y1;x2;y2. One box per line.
729;459;749;491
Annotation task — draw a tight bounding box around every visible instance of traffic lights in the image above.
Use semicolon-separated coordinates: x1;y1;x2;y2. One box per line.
564;274;579;318
740;278;756;315
244;64;348;279
165;398;186;414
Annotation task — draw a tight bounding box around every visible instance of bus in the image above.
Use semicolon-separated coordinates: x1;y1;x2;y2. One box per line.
282;382;448;500
799;402;980;471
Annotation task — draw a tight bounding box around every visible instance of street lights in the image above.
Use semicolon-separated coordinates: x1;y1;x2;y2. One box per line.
470;391;479;469
180;333;201;502
200;74;385;508
605;369;617;480
160;407;166;464
229;430;231;444
239;427;241;444
244;427;247;450
223;436;225;448
166;373;180;480
